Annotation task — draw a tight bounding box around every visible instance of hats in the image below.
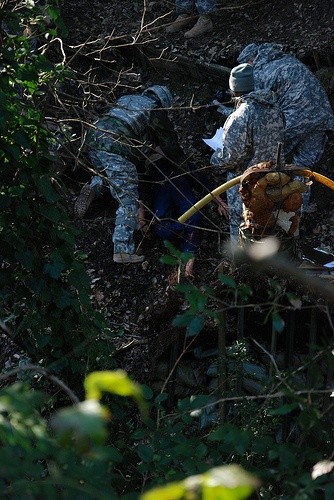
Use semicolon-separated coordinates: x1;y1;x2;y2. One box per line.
237;43;258;63
229;63;254;91
141;85;174;114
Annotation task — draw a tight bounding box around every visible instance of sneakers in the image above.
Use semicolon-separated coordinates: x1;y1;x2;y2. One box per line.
74;186;95;218
113;253;144;262
184;15;213;38
166;14;194;32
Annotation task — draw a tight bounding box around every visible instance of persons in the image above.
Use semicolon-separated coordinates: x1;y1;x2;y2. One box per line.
74;84;188;263
166;0;214;38
139;164;229;278
210;63;285;260
237;43;334;217
